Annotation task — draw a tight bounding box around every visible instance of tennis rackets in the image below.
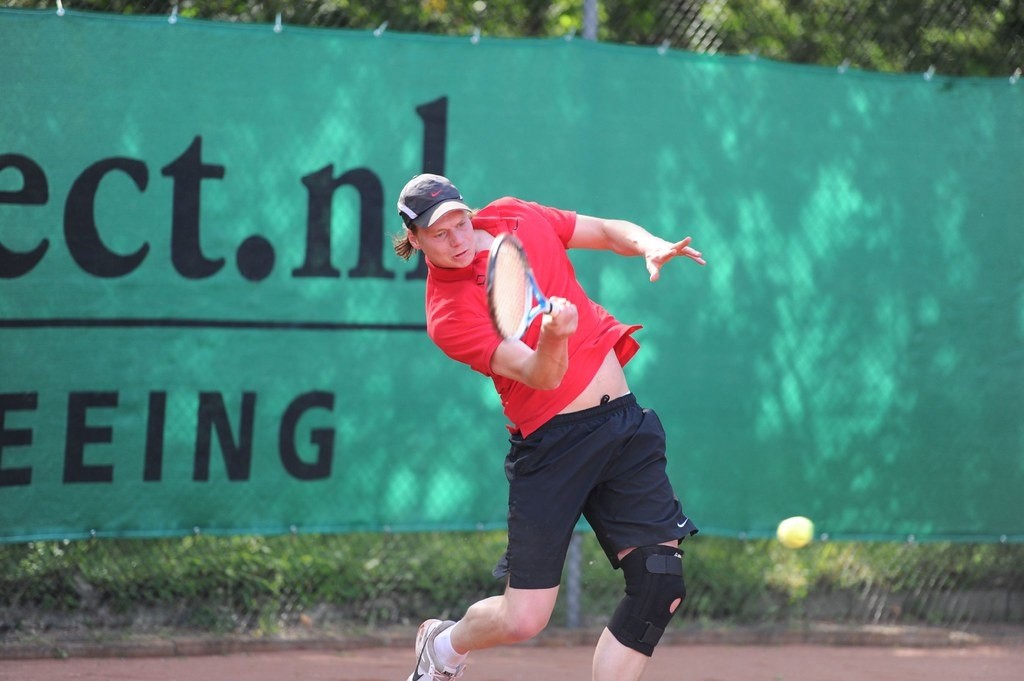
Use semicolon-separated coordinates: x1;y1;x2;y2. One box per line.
484;234;558;347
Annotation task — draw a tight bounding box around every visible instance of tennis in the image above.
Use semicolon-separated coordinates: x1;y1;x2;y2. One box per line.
776;516;814;549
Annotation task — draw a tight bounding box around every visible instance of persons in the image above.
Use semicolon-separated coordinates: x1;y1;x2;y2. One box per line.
393;173;708;681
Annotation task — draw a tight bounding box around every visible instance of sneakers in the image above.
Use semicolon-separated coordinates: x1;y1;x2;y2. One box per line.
407;618;466;681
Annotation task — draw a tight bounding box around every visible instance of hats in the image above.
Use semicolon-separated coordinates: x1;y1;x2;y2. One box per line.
397;173;473;229
